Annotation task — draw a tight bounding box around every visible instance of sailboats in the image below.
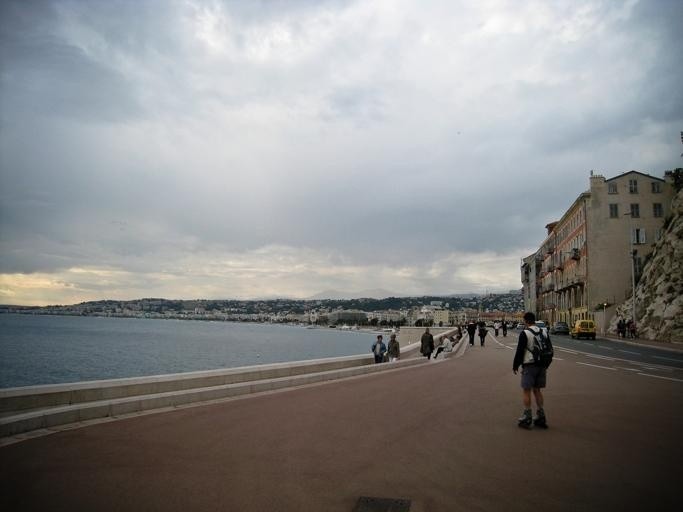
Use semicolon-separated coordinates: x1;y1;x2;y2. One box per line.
278;318;399;336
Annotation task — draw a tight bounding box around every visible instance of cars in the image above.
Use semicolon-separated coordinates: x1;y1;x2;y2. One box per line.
487;320;526;330
550;322;569;334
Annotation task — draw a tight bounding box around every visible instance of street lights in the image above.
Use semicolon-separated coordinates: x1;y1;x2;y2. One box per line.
623;211;637;331
603;302;607;336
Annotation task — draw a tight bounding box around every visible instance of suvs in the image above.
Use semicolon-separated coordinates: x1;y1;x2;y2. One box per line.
535;321;547;333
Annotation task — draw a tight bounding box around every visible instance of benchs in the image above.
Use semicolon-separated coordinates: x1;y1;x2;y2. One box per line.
441;330;468;358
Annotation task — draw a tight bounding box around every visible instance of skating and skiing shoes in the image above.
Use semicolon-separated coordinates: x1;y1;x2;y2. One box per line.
518;409;532;431
533;410;550;430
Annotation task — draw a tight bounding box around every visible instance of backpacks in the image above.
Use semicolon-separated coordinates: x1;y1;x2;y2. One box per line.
526;328;553;371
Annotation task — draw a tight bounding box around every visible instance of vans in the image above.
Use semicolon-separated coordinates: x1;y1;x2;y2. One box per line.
570;319;597;340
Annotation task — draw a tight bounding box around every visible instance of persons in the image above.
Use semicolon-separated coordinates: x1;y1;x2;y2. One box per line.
372;335;386;363
419;318;520;360
512;312;553;429
544;321;549;331
386;334;400;362
617;319;636;340
